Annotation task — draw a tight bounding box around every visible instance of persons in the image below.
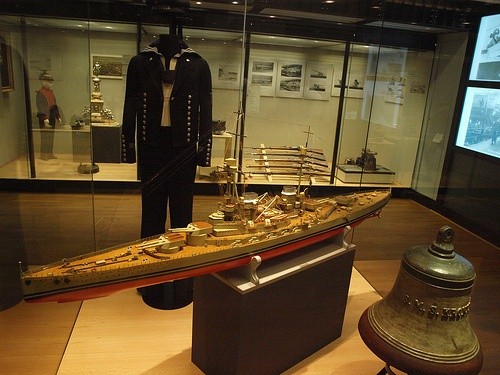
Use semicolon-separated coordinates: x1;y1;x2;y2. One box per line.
36;73;62;161
121;33;213;298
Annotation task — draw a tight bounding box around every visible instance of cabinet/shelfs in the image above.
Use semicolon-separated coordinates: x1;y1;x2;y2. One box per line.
197;132;232;180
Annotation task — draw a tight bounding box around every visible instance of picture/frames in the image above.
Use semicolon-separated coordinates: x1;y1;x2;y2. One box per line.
90;54;123;80
0;30;15;95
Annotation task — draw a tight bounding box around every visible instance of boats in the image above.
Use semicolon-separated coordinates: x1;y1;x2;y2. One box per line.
19;99;392;304
